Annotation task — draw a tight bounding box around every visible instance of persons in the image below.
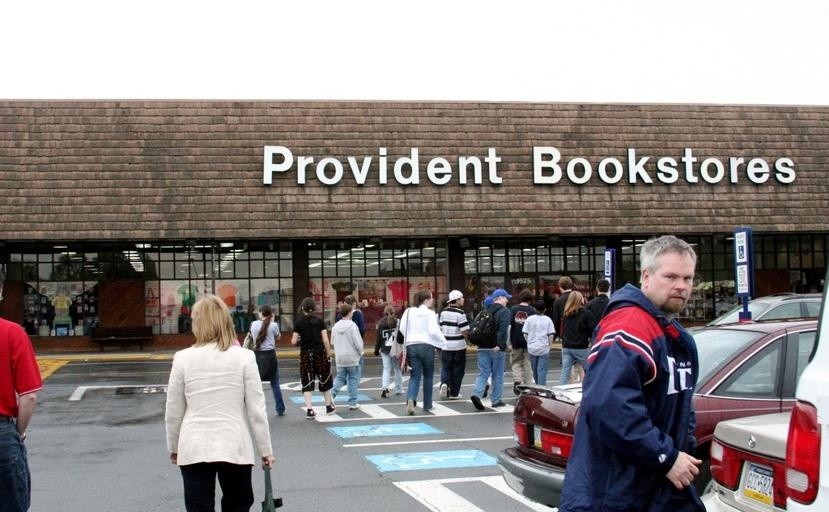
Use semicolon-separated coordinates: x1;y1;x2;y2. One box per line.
557;234;708;510
375;277;611;416
291;295;365;418
0;316;44;511
163;294;278;512
243;305;285;416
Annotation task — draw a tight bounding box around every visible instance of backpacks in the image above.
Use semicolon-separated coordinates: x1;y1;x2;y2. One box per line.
467;304;502;348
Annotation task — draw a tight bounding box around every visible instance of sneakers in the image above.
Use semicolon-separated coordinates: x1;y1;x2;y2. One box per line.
440;383;448;398
514;381;520;395
425;404;435;410
471;395;485;410
396;390;405;395
279;410;283;416
483;383;489;397
407;399;414;415
381;390;386;397
492;400;505;407
349;403;360;409
448;394;462;399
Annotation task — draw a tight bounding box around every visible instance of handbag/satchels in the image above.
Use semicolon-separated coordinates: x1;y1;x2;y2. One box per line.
389;340;407;374
242;332;253;350
262;494;276;512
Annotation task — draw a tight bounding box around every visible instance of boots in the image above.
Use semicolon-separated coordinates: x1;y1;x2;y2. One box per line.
326;405;336;414
306;409;316;418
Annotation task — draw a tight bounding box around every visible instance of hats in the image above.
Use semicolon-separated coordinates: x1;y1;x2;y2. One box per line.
484;289;513;306
447;290;463;304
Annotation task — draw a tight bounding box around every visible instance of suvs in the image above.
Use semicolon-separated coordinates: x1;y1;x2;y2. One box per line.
782;259;829;512
680;291;823;398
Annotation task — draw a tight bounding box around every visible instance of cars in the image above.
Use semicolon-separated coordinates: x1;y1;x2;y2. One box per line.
496;314;821;511
697;407;792;512
